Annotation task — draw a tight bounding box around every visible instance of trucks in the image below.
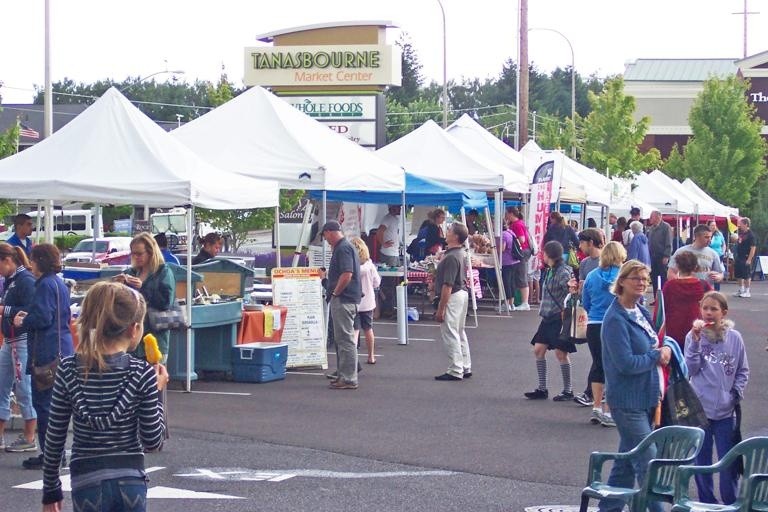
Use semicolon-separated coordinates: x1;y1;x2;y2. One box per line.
150;207;202;253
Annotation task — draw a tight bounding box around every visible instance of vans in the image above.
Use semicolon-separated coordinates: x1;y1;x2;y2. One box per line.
61;236;134;266
0;208;103;241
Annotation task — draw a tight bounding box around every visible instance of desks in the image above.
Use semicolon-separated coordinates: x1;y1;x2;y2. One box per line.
373;249;521;321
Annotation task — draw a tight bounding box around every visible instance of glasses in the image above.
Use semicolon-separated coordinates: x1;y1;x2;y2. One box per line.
622;276;649;284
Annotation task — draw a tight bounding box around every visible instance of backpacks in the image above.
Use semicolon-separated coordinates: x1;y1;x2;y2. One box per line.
505;230;526;260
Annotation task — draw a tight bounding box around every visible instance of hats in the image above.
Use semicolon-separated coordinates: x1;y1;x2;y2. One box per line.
317;219;341;236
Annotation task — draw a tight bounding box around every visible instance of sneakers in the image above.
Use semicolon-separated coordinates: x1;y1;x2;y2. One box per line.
524;388;618;427
494;303;530;311
326;370;359;389
5;432;38;452
731;289;751;298
435;373;472;380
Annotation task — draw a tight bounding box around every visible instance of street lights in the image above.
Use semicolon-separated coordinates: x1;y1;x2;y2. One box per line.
119;70;185;93
524;25;577;162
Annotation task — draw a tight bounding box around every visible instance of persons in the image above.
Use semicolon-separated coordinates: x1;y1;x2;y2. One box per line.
194;233;221;264
426;207;472;381
734;218;757;297
599;258;749;507
319;220;381;388
524;207;749;427
464;207;531;310
377;205;402;310
1;214;177;510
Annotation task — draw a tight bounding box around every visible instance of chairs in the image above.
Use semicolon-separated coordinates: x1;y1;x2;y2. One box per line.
578;424;705;512
670;437;767;511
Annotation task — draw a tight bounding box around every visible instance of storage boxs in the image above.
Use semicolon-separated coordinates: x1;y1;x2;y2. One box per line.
229;341;288;383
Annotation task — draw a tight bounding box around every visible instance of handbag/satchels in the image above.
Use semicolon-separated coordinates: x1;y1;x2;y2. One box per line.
733;424;745;478
660;378;711;430
527;230;539;256
143;298;189;333
558;306;588;344
567;248;580;271
32;357;61;393
